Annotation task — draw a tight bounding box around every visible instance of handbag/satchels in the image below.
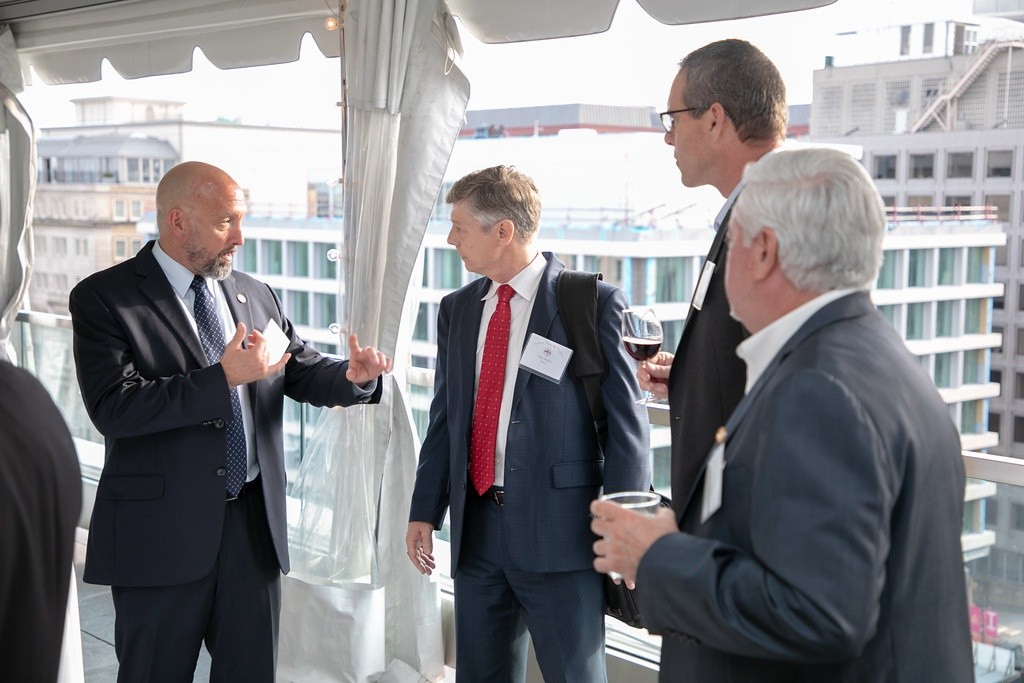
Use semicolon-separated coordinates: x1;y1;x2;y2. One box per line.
595;487;672;629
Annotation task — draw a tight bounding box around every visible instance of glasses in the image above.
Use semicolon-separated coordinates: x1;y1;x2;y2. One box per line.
659;107;735;134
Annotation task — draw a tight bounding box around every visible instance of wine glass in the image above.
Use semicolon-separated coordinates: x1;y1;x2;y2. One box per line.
621;307;667;406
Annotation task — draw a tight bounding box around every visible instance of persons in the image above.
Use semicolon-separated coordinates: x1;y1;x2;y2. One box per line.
591;148;976;683
0;358;83;683
406;164;652;683
68;161;393;683
634;38;790;502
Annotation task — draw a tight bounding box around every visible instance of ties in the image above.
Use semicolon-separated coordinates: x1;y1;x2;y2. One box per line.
469;283;516;495
190;274;248;499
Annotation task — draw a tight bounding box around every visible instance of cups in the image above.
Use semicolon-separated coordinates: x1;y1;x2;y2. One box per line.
601;492;661;579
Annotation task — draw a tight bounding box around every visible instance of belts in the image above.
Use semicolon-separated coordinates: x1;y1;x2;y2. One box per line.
224;478;265;502
468;484;503;505
